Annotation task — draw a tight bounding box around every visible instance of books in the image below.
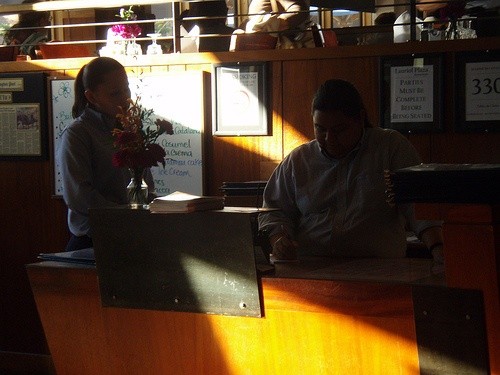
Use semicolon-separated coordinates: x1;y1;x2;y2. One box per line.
148;191;226;213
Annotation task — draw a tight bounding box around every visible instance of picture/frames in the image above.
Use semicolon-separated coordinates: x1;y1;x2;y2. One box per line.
453;48;500;133
380;54;446;136
211;61;269;136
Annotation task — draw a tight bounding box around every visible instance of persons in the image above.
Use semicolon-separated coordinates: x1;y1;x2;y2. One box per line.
256;80;450;273
3;0;500;57
61;57;154;251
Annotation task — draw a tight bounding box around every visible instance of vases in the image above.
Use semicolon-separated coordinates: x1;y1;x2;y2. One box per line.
126;178;149;210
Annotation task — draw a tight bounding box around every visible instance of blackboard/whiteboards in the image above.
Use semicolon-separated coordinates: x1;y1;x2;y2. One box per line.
47;72;207;203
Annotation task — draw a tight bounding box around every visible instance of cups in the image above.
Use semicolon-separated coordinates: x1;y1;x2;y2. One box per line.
445;18;454;40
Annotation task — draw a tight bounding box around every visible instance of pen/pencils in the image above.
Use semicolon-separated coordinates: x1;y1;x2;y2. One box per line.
281;224;297;259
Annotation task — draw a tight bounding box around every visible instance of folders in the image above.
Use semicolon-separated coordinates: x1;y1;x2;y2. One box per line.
38;249;97;266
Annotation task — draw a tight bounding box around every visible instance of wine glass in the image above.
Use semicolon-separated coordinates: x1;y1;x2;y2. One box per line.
465;17;477;39
146;33;162;54
127;33;142;55
456;18;467;39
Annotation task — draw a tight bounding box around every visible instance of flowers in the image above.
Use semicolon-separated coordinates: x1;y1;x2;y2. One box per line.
111;5;142;38
111;92;174;200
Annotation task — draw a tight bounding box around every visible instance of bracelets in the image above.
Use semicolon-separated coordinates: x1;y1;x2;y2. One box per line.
428;243;444;254
